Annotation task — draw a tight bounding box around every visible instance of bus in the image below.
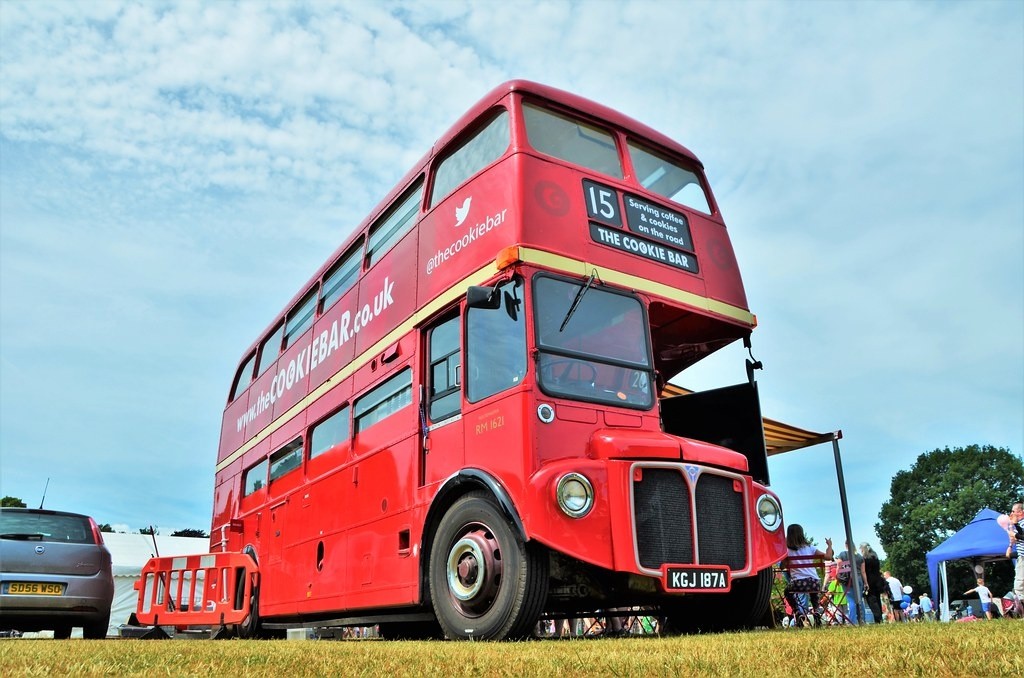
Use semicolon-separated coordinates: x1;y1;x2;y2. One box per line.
209;79;789;642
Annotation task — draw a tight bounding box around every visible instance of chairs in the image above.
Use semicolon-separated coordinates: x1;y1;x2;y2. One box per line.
780;554;858;628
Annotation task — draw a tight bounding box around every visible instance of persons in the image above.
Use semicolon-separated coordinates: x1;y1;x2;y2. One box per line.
961;502;1024;621
768;524;934;629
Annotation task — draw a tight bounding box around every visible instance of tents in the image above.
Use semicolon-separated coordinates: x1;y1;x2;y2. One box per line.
24;531;212;640
926;507;1018;623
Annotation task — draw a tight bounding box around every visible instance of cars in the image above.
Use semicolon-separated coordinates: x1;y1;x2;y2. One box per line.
1;508;115;642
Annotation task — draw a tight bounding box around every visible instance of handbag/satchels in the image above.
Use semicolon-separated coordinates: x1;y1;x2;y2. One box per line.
836;560;851;586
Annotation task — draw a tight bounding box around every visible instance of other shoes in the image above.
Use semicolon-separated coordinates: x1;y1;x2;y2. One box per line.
813;612;822;625
795;611;805;626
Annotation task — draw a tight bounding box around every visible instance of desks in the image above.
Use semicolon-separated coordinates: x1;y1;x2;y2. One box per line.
771;568;812;628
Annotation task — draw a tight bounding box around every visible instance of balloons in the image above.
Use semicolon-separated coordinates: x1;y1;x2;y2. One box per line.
900;602;909;609
902;595;911;603
903;586;913;594
